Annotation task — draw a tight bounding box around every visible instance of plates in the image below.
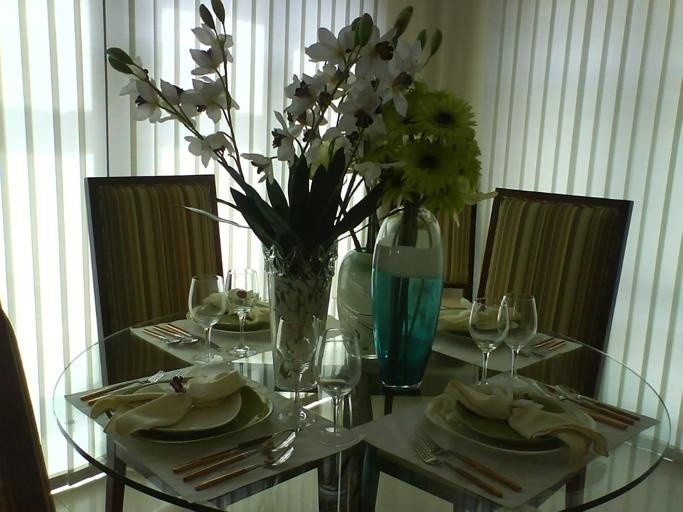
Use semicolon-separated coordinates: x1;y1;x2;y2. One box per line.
423;383;594;456
186;306;278;337
99;363;270;446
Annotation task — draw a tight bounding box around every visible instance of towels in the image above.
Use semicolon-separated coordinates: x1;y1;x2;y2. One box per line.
86;358;246;439
434;295;522;335
442;367;610;469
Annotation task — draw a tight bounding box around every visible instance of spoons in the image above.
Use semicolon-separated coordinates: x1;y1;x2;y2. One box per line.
541;383;641;429
516;337;567;360
168;428;298;490
140;324;219;350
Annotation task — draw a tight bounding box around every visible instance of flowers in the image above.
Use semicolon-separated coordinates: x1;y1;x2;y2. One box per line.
107;1;482;243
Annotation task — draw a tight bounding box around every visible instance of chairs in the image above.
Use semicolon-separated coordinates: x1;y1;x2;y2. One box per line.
363;202;477;414
353;187;638;510
80;173;341;511
0;301;213;511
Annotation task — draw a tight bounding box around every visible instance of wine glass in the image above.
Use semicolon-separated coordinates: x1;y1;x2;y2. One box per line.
468;291;538;393
276;314;362;448
186;269;258;368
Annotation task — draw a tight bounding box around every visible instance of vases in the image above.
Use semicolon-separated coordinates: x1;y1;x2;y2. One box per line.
370;202;444;393
263;243;339;394
336;248;373;356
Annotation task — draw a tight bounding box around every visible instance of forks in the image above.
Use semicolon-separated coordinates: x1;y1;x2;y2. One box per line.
79;370;182;406
407;428;520;498
